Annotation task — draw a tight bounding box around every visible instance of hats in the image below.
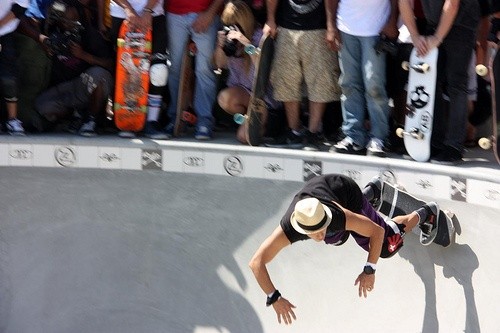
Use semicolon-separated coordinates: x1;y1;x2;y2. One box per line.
290;198;333;235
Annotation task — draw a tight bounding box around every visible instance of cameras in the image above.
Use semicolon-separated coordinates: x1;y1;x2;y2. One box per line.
42;10;84;64
219;22;243;57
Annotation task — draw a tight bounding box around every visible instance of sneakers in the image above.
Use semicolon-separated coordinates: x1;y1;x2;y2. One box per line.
67;117;81;133
194;126;212;139
365;177;384;211
78;122;96;137
419;202;439;246
263;126;302;148
430;144;464;165
117;129;136;138
365;138;386;158
302;129;331;151
141;128;168;139
161;122;175;138
328;136;366;155
5;118;25;136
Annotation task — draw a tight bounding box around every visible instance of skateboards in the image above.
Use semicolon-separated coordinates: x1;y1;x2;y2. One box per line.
172;31;197;137
234;31;276;146
395;45;439;161
373;174;455;248
113;16;152;131
474;38;500;161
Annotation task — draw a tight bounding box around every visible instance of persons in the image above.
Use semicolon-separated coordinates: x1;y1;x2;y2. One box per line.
249;173;440;325
325;1;396;157
215;0;267;147
1;1;113;138
263;1;337;149
104;1;170;139
401;1;474;165
165;0;223;141
